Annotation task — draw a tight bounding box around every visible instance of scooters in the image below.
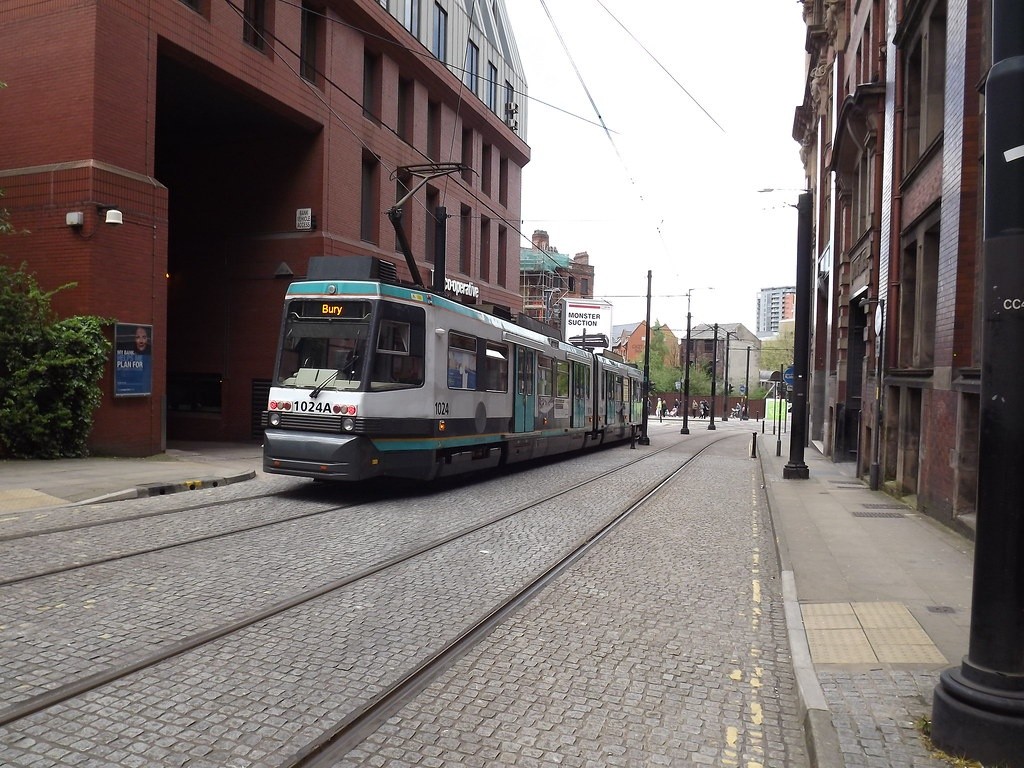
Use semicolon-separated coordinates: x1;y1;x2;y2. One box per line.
729;407;740;419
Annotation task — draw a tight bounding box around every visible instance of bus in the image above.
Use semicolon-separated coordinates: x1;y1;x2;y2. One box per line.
260;162;645;487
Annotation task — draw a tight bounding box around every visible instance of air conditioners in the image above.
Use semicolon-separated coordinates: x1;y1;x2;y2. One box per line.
509;119;519;130
508;102;518;114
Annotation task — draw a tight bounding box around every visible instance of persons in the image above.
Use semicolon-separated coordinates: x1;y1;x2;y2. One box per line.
647;397;652;415
735;402;749;420
674;399;680;416
134;327;148;355
656;398;667;417
692;399;709;419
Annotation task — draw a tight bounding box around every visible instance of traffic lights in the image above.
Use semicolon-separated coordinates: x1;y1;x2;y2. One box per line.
728;384;733;395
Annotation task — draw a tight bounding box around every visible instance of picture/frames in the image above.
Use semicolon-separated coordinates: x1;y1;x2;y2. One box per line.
114;321;153;398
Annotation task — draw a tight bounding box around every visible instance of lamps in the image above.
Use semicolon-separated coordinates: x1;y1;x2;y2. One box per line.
96;203;124;225
